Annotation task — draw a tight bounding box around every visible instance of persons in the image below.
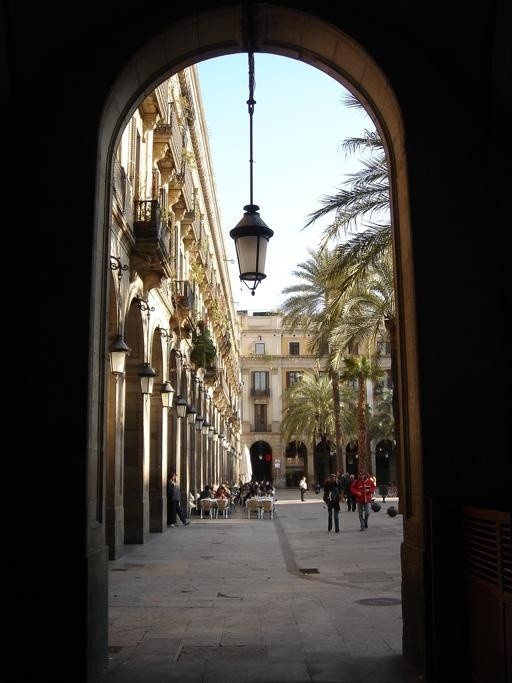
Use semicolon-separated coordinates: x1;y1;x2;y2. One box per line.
349;468;376;531
167;471;192;527
323;473;340;532
334;468;391;512
298;475;308;502
189;479;279;516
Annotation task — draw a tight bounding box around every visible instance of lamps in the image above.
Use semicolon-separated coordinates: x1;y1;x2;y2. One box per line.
109;254;244;461
229;51;276;295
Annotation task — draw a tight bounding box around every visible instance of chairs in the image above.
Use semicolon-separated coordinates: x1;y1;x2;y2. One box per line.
197;487;276;519
